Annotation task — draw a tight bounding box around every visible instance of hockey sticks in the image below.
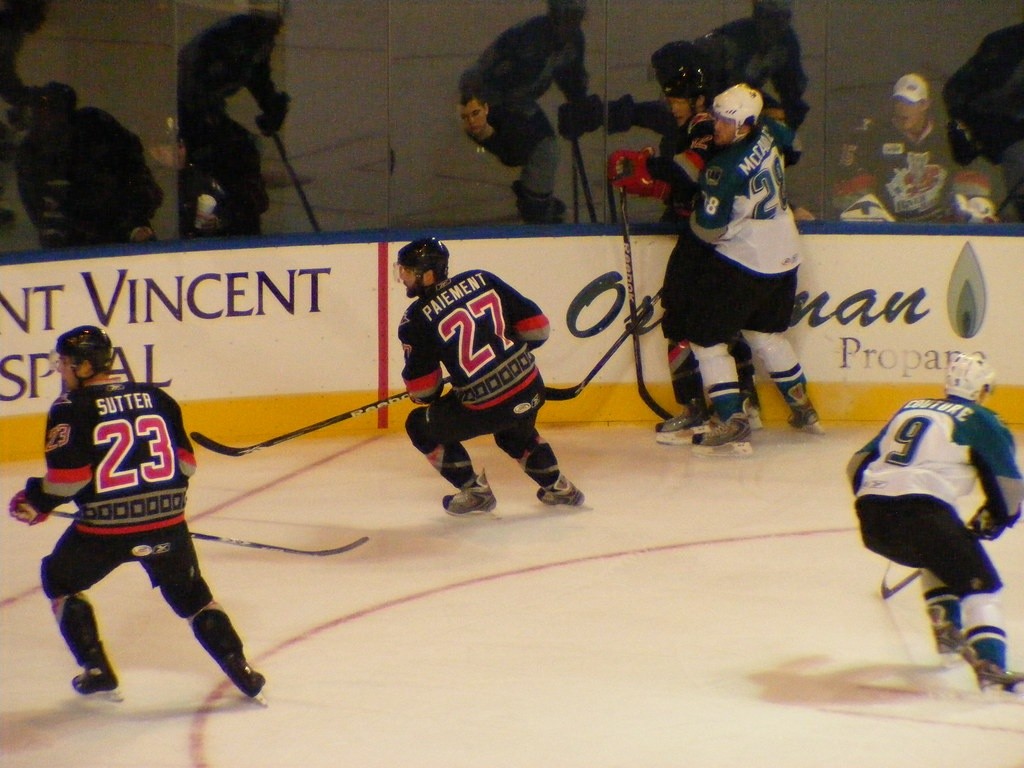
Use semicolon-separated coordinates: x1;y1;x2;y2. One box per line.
568;137;598;226
51;508;372;559
189;377;452;458
270;132;325;233
541;287;667;402
619;184;674;421
877;560;925;599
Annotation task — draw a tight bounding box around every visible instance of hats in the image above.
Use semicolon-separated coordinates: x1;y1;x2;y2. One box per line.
892;73;928;103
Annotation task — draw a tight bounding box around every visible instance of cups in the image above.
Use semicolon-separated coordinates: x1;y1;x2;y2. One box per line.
195;196;217;230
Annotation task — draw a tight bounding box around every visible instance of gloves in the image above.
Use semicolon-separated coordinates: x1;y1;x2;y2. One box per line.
255;105;290;136
9;489;49;526
607;146;673;205
972;507;1007;539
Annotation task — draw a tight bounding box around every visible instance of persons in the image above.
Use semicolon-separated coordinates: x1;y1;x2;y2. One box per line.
0;0;50;223
17;82;166;248
847;353;1024;689
7;326;266;698
652;0;810;157
834;74;1002;222
942;23;1024;222
396;241;585;516
456;0;606;224
608;64;820;447
176;0;291;237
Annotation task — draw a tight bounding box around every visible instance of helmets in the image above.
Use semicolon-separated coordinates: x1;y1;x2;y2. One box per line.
946;353;992;401
712;83;764;138
398;235;450;298
56;325;111;375
661;63;705;99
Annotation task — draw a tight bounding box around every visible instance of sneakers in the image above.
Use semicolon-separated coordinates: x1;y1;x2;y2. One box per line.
692;398;752;457
962;646;1024;695
655;390;711;445
512;180;567;222
787;397;824;435
218;648;270;707
537;473;593;510
443;467;501;519
72;642;124;703
926;605;963;654
